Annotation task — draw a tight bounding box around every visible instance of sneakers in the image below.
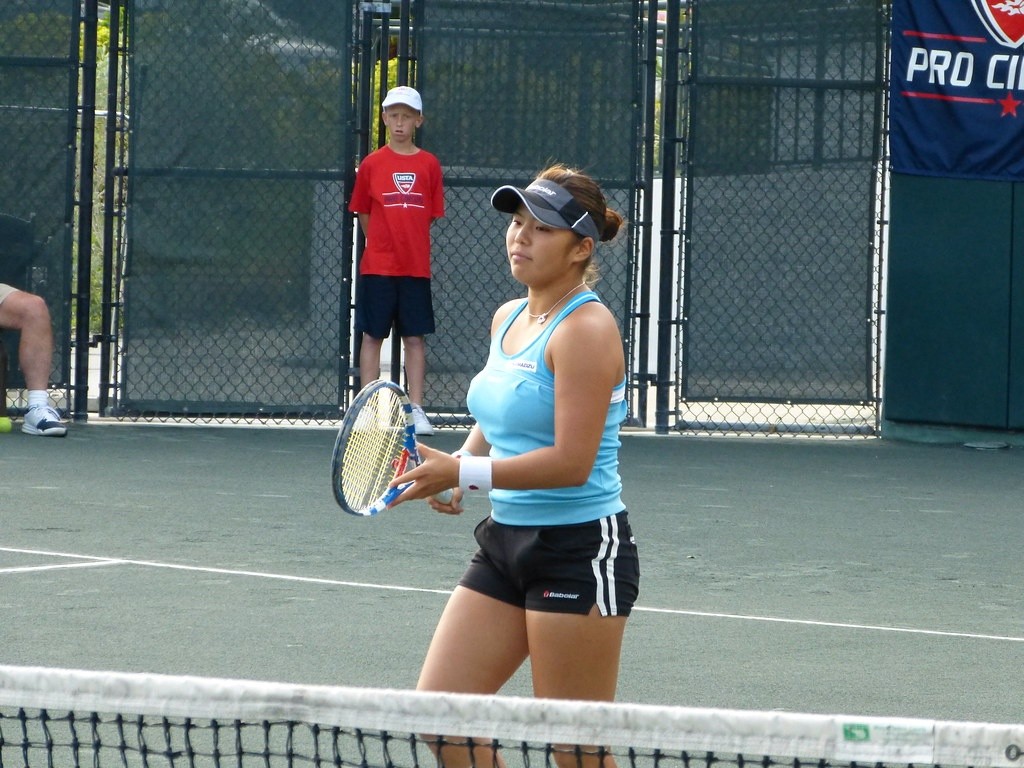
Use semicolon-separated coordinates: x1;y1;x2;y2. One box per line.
349;405;377;434
403;403;435;435
21;406;68;435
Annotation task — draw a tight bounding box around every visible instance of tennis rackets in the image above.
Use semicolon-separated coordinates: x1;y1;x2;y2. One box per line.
331;380;469;518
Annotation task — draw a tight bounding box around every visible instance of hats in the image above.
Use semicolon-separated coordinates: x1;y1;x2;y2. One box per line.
382;86;422;116
490;178;600;243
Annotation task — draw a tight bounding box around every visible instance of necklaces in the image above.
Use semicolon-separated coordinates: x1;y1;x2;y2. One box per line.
527;281;586;324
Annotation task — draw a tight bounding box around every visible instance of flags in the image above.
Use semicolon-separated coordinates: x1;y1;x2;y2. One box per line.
889;0;1024;183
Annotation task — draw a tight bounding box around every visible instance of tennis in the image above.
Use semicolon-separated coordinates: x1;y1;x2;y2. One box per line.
0;417;13;433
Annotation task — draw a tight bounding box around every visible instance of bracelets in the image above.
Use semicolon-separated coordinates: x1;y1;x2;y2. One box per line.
459;456;492;497
450;450;472;461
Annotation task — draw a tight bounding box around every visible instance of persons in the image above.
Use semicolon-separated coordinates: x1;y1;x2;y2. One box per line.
0;284;67;436
348;86;444;436
386;165;641;768
